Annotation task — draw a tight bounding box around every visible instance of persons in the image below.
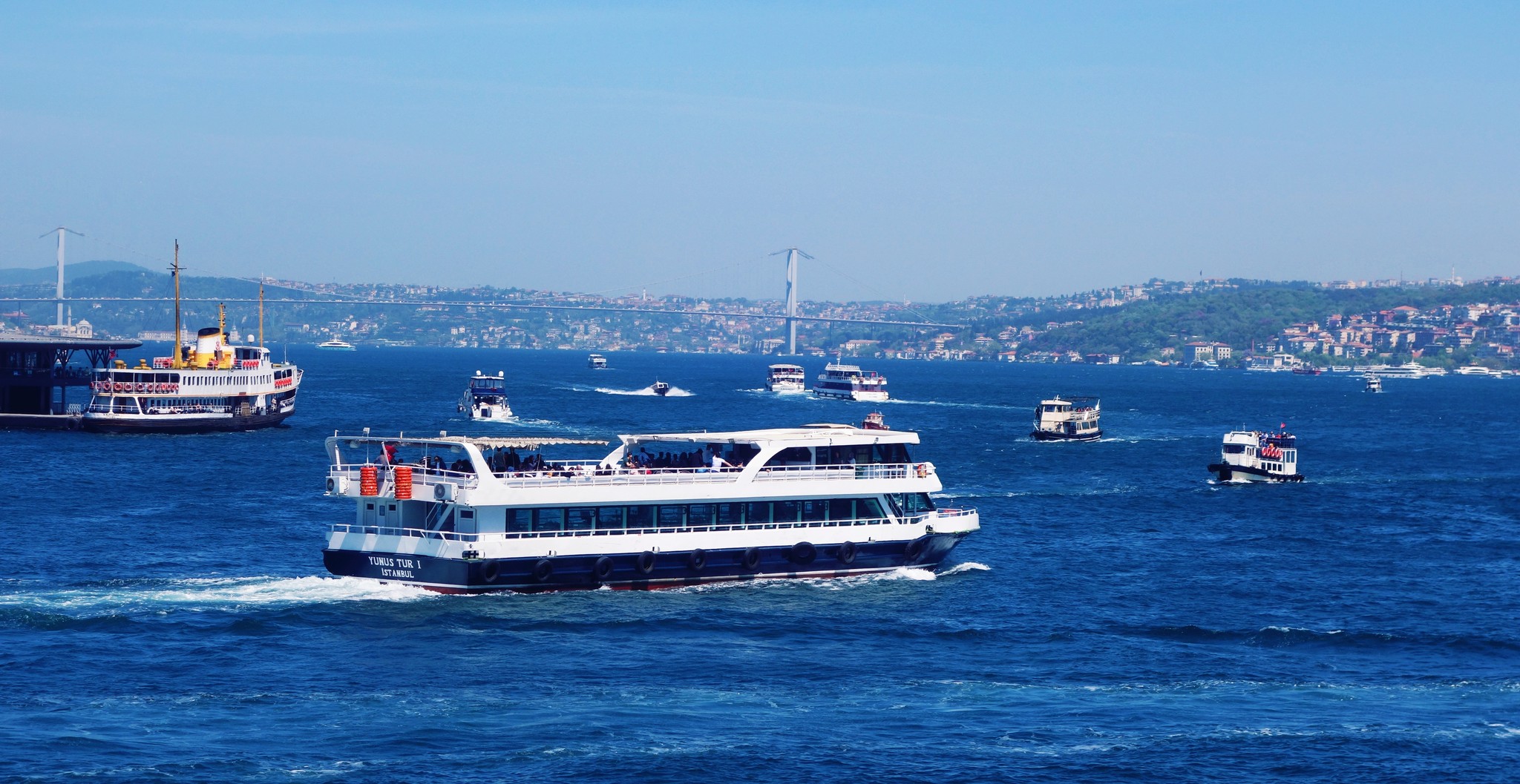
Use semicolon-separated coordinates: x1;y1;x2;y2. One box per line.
1069;406;1073;411
1056;422;1062;432
603;464;611;475
846;452;856;469
879;375;883;381
620;444;746;474
1075;406;1092;412
272;397;277;411
1250;431;1291;447
852;374;855;379
776;460;789;471
759;464;770;472
106;376;111;384
430;455;447;476
373;449;397;470
55;366;91;379
169;403;212;414
832;451;843;469
593;465;603;476
398;459;403;466
1035;407;1040;420
449;447;586;477
1047;406;1053;412
767;378;770;381
141;397;146;413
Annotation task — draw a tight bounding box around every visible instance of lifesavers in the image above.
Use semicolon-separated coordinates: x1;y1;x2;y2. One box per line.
689;548;707;573
89;382;179;392
1261;446;1283;458
765;382;772;390
838;541;857;564
532;559;553;582
275;377;292;386
745;547;763;571
791;542;817;565
243;361;259;367
394;467;412;499
593;556;613;583
480;558;501;583
904;539;923;561
360;467;378;496
917;462;927;477
798;386;800;389
776;374;779;378
637;550;656;574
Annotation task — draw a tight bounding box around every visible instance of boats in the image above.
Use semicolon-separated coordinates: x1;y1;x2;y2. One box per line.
1362;362;1430;379
319;408;983;597
587;354;607;369
456;370;513;419
1190;360;1221;370
649;376;669;397
1361;376;1383;393
78;237;304;435
860;411;891;431
1292;366;1321;375
1207;425;1305;487
1028;393;1103;443
314;339;356;351
765;364;805;394
812;349;889;401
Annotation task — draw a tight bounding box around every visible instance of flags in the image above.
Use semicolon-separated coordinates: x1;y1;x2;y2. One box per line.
1281;423;1285;428
110;349;116;360
